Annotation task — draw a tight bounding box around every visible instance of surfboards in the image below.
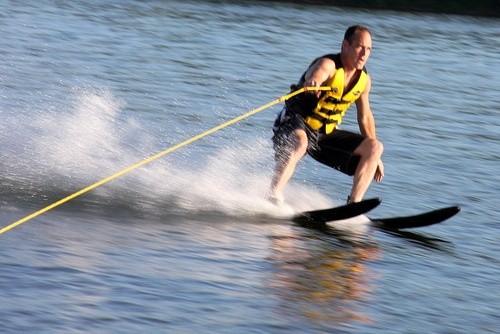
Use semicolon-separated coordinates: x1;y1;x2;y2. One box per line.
365;203;461;233
282;192;381;238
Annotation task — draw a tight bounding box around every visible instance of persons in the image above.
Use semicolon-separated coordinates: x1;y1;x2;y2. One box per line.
260;24;386;208
